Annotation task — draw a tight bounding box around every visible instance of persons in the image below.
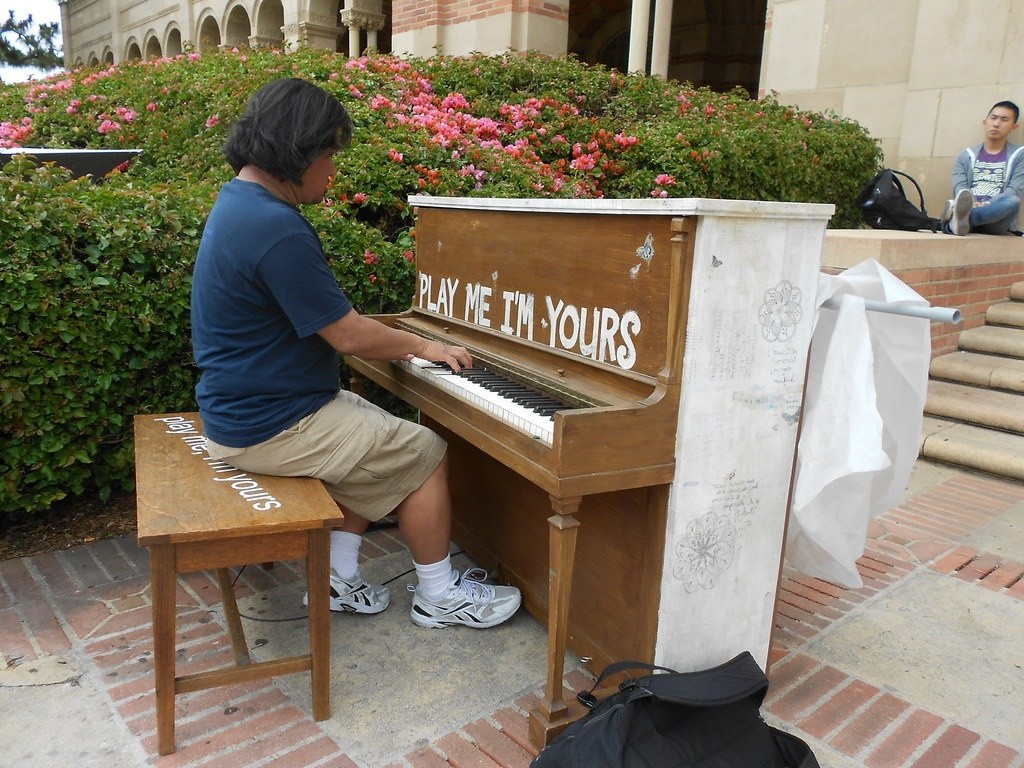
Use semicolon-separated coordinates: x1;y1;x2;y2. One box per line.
941;100;1024;236
192;78;522;629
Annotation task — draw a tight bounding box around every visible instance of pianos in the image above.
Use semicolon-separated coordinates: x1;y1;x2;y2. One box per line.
343;195;839;753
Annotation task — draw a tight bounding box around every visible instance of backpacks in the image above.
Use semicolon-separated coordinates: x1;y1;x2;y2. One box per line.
858;169;929;232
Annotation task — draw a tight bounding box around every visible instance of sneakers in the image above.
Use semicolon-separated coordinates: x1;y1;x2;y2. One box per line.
406;567;522;629
302;567;391;614
948;189;973;236
941;199;954;222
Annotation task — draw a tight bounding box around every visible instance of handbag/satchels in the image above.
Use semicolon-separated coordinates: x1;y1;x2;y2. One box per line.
529;651;821;768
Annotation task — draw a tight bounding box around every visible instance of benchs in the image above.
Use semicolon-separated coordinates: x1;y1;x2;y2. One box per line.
133;411;345;755
